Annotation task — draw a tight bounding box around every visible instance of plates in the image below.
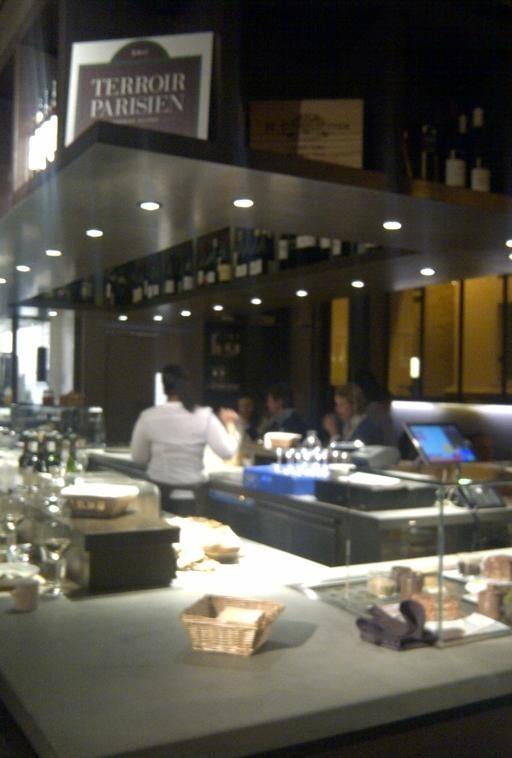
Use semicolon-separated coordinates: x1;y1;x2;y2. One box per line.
0;561;41;588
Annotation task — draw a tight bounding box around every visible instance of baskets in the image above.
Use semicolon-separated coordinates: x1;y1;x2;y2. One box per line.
180;593;288;659
59;483;143;518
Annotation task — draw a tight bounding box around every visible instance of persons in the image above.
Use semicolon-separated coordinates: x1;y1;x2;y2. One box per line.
301;382;384;449
130;362;241;518
234;384;303;440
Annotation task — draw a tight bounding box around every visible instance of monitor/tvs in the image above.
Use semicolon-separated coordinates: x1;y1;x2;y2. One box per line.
402;420;476;467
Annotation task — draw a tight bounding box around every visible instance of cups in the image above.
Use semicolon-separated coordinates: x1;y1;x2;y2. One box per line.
8;579;39;612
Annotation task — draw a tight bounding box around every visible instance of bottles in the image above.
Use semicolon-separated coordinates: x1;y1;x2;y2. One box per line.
19;429;83;478
57;227;344;304
444;107;497;193
